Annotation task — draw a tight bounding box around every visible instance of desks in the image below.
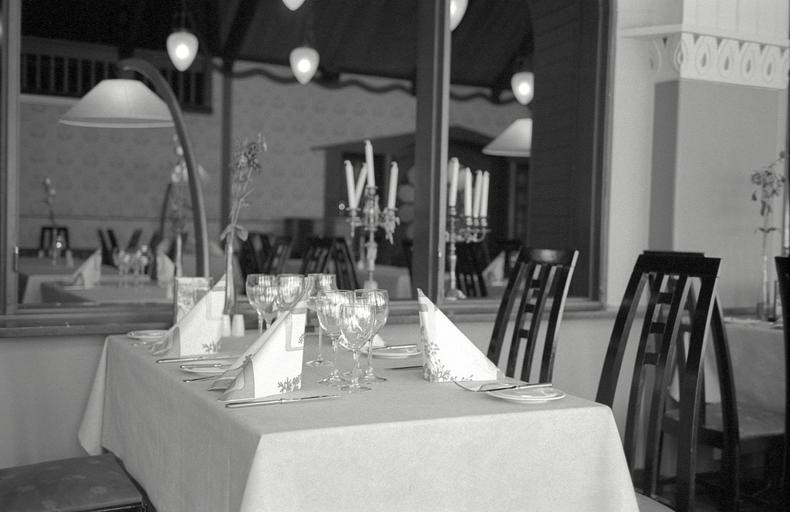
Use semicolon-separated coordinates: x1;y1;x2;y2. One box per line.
651;308;790;511
79;323;642;512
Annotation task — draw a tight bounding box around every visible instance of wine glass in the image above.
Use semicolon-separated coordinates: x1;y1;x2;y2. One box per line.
246;271;391;393
113;242;155;289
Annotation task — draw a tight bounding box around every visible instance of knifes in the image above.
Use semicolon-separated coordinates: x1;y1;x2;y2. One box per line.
155;352;243;365
384;364;425;370
180;362;234;370
224;394;342;410
477;381;554;393
373;343;419;351
185;375;220;383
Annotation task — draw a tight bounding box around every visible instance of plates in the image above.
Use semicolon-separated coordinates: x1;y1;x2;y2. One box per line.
361;344;424;358
485;382;565;404
180;356;231;374
126;329;169;338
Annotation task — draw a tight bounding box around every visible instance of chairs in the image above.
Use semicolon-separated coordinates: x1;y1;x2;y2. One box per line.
1;452;145;512
641;249;790;512
0;228;526;316
486;244;578;390
594;252;722;510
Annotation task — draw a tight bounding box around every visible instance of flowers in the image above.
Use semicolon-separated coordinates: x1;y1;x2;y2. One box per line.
750;152;788;255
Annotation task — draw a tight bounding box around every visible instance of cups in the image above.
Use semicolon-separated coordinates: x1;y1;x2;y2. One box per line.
231;314;245;339
219;315;231;338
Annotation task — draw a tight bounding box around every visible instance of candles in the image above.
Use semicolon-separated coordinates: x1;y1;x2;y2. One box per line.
448;157;491;219
343;139;400;213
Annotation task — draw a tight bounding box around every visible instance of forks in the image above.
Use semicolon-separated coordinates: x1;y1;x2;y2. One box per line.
450;378;520;391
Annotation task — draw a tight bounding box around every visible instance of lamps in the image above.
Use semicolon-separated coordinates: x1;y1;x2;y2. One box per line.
58;55;210;275
166;0;199;69
481;117;534;157
510;44;535;107
289;16;320;86
